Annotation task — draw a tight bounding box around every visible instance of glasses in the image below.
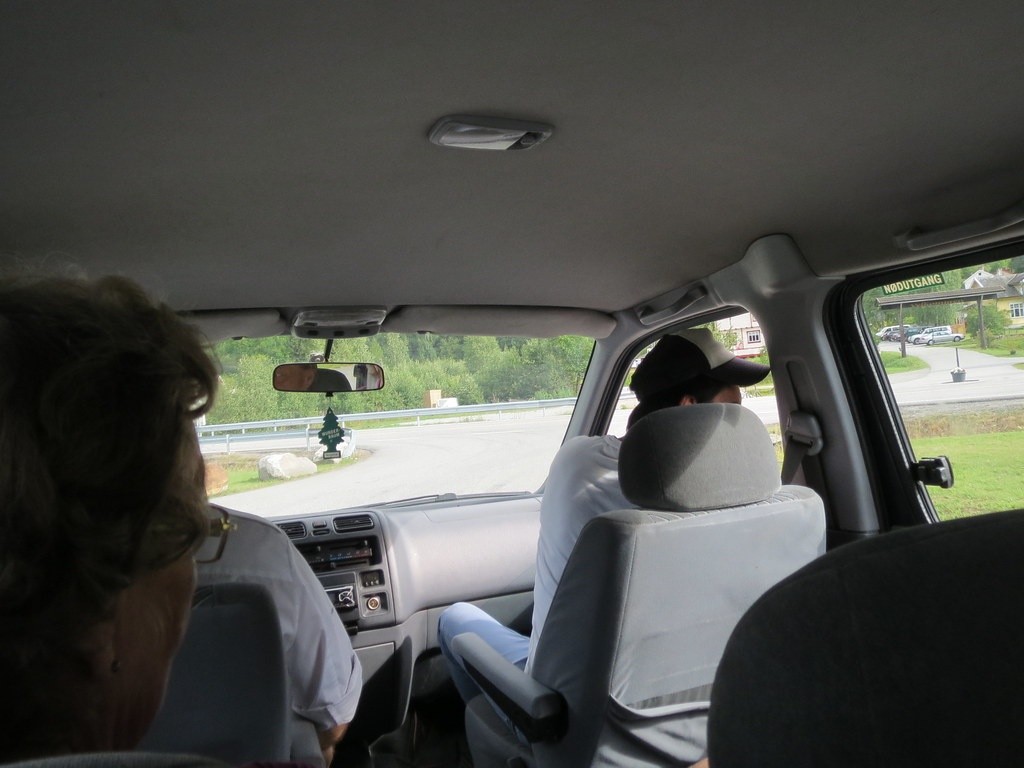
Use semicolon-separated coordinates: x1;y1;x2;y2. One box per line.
193;504;240;565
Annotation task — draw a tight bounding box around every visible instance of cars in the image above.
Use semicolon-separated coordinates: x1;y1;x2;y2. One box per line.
876;325;966;344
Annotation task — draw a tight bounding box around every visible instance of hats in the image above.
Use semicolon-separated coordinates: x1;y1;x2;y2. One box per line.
629;328;770;392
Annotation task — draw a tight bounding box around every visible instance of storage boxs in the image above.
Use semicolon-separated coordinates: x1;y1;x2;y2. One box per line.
425;389;456;407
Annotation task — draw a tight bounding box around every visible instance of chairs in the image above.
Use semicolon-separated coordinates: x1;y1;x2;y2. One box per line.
132;580;327;768
704;510;1024;768
454;399;825;767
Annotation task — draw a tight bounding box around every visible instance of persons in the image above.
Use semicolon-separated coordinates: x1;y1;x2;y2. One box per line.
274;363;316;392
0;271;238;760
195;502;363;768
437;328;773;749
